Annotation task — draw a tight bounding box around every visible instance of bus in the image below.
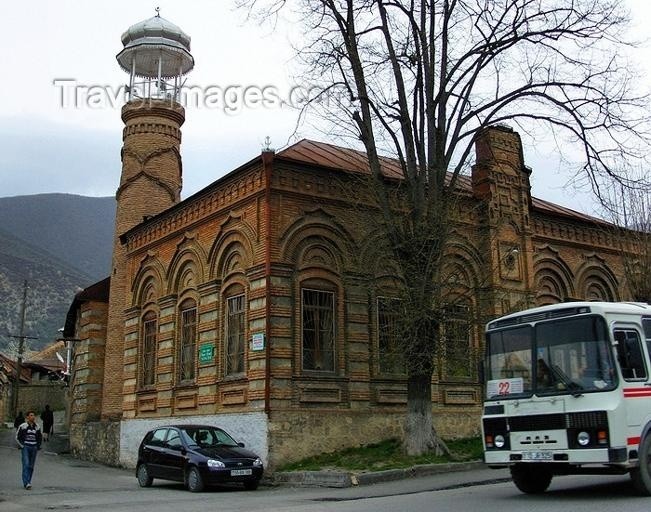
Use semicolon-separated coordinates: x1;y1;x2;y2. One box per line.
474;298;650;492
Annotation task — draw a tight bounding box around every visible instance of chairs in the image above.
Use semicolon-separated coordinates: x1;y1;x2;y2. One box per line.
196;431;210;449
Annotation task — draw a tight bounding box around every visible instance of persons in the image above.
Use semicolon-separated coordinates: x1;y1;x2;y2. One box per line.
40;405;54;443
500;353;530;391
15;411;42;490
14;411;25;430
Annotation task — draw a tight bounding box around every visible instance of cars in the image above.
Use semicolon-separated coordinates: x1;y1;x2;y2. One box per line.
135;425;265;491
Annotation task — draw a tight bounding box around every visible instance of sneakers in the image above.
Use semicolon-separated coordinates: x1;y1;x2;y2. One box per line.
24;483;32;489
